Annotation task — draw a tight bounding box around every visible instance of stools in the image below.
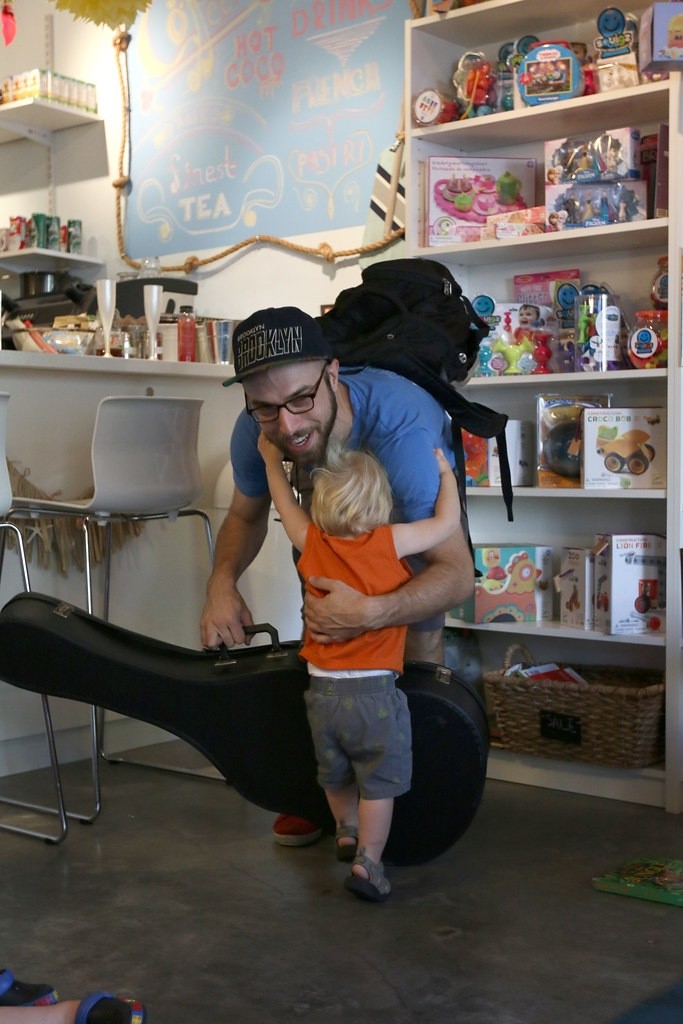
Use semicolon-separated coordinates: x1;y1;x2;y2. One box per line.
1;394;69;845
12;396;215;825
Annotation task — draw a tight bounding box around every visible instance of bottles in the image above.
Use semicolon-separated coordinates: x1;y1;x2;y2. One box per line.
627;311;668;368
178;305;197;363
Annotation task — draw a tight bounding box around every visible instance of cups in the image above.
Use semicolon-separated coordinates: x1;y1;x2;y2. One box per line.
497;171;523;205
574;294;626;372
214;320;235;365
142;256;160;277
447;173;496;211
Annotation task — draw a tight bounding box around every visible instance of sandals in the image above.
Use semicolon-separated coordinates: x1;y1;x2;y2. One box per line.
334;817;358;862
345;847;391;902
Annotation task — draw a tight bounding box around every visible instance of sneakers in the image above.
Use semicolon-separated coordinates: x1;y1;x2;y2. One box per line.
271;810;323;847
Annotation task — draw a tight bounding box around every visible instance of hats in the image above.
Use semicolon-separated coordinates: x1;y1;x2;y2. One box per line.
220;307;330;386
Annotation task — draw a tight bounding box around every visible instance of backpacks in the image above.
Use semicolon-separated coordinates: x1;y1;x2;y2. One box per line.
314;258;508;439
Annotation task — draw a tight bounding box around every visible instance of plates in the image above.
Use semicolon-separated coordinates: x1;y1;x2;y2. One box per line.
441;184;500;215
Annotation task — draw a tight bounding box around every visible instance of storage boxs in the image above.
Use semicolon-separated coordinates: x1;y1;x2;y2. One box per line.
637;0;683;74
423;125;673;247
445;529;665;637
456;393;665;488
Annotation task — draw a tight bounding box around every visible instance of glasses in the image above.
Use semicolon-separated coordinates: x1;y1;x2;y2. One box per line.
245;362;328;424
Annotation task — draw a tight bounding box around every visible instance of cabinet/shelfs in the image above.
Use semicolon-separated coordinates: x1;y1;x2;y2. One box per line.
0;96;109;271
406;0;683;814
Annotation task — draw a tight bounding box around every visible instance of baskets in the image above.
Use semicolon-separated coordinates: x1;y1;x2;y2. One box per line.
484;642;668;769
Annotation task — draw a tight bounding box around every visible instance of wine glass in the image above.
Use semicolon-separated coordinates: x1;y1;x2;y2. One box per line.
143;284;163;361
96;279;117;358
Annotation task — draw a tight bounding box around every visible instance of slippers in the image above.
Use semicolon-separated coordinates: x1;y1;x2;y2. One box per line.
0;968;59;1007
74;992;147;1024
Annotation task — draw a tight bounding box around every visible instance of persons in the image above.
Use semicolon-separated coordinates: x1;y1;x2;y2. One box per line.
258;430;461;903
563;146;617;170
566;192;628;225
0;970;147;1024
507;305;540;345
200;307;475;846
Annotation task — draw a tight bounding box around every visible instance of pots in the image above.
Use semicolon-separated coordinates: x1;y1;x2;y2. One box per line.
20;271;68;297
